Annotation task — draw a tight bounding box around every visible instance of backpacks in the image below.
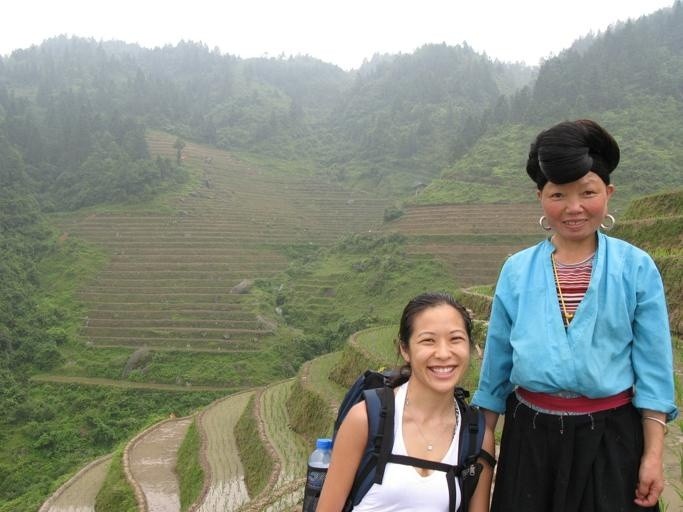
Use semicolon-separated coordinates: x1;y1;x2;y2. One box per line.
333;368;497;511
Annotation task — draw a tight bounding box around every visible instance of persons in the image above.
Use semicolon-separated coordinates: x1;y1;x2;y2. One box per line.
314;291;495;512
472;120;677;512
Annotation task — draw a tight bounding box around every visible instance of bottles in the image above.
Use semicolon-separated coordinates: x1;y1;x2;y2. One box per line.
304;438;334;512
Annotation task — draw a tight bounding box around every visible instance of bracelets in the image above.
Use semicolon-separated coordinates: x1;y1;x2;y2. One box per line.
642;415;668;436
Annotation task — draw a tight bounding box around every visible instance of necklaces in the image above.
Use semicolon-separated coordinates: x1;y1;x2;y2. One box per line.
406;395;451;451
